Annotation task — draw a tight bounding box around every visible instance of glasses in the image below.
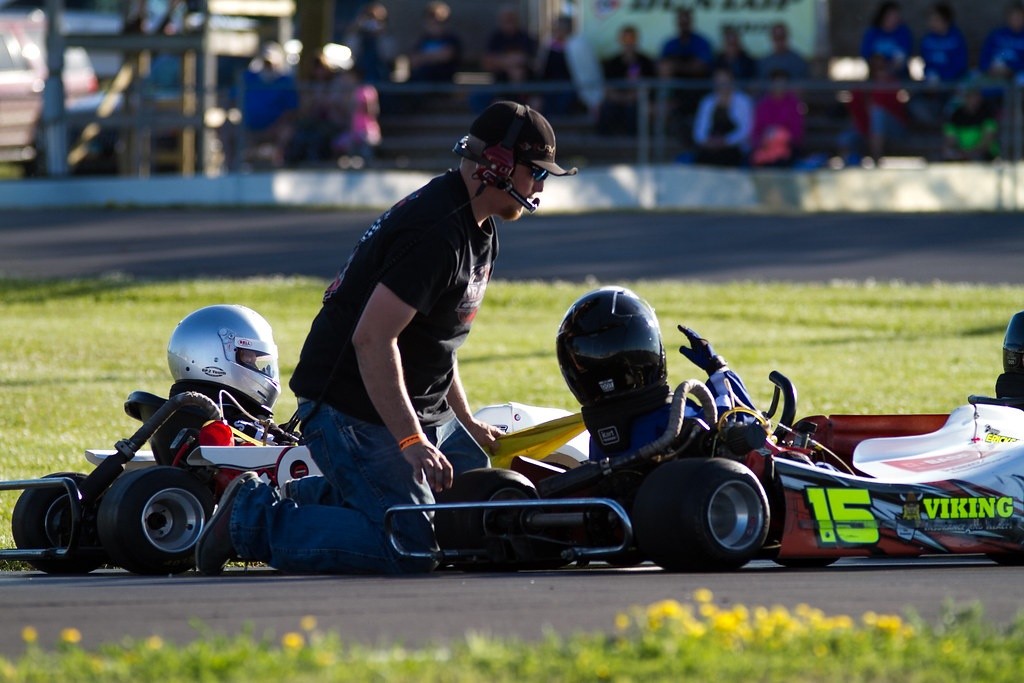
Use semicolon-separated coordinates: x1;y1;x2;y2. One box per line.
520;162;548;182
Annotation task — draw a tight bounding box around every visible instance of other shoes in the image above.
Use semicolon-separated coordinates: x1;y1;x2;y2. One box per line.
194;470;259;574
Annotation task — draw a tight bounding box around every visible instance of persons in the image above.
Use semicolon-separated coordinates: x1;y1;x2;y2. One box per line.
556;286;770;463
194;101;578;576
1003;311;1024;372
167;304;300;446
231;0;1023;168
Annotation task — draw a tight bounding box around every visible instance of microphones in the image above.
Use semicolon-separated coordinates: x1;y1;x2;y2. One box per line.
483;170;540;214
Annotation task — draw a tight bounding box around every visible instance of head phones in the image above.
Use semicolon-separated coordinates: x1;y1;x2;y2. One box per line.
476;103;525;179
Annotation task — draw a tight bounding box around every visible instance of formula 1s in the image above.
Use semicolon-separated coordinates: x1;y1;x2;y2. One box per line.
384;370;1024;573
0;391;597;578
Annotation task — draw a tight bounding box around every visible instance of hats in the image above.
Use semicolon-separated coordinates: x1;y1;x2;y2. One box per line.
468;101;578;178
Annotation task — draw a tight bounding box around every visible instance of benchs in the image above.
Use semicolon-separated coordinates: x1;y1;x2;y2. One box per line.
299;61;1024;173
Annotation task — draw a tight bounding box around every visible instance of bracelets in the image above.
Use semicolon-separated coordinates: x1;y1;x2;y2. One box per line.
400;434;427;450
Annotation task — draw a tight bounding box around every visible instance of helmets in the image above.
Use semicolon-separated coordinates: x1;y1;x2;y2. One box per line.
555;286;669;405
166;304;280;419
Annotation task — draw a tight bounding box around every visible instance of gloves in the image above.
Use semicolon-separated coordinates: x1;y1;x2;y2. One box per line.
677;323;728;376
